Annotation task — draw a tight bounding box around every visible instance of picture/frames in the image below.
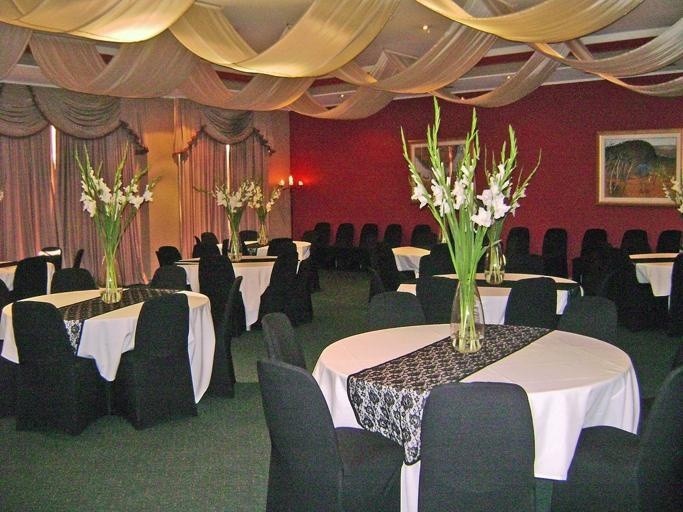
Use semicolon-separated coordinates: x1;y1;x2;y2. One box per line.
596;128;681;207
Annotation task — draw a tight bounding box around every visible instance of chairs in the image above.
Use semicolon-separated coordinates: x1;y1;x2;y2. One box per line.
0;214;682;512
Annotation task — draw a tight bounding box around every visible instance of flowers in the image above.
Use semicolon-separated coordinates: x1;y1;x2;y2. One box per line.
245;180;284;245
73;140;162;304
405;101;544;353
192;176;259;261
478;141;541;283
